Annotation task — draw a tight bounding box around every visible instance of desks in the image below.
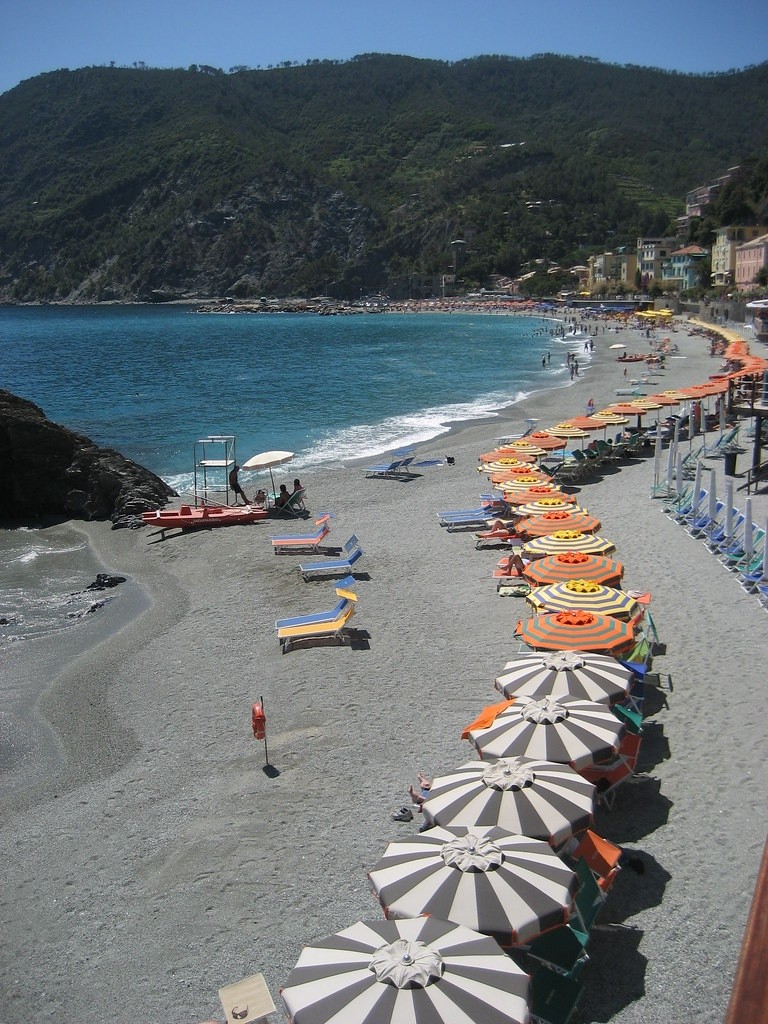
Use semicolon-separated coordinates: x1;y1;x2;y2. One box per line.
215;970;278;1024
390;448;418;464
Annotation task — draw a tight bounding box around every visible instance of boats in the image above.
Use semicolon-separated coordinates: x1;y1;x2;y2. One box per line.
142;500;269;528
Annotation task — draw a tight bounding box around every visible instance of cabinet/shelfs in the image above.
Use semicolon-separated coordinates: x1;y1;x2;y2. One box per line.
194;434;238;507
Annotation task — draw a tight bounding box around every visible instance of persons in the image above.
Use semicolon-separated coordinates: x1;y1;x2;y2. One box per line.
273;484;290;507
475;519;516;538
541;316;679;380
407;770;433;804
500;554;526;577
290;479;304;503
229;465;253;505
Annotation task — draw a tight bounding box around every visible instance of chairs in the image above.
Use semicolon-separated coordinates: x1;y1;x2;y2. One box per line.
273;488;364;655
360;455;418;481
436;364;768;1024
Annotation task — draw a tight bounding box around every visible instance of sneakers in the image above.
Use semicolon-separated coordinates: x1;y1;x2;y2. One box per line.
393;807;413;821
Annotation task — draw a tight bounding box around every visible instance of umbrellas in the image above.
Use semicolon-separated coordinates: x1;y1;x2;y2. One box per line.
416;756;597;847
563;319;768;431
526;579;642;625
242;450;295;499
476;424;602;534
495;649;635;708
460;694;629;772
522;530;616;557
515;608;635;656
280;913;531;1024
521;553;625;587
367;825;577;947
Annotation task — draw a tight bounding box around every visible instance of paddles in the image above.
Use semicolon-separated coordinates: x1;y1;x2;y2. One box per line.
181;491;230;509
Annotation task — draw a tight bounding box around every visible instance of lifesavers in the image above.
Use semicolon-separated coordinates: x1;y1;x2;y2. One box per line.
623;367;627;376
251;703;266;740
